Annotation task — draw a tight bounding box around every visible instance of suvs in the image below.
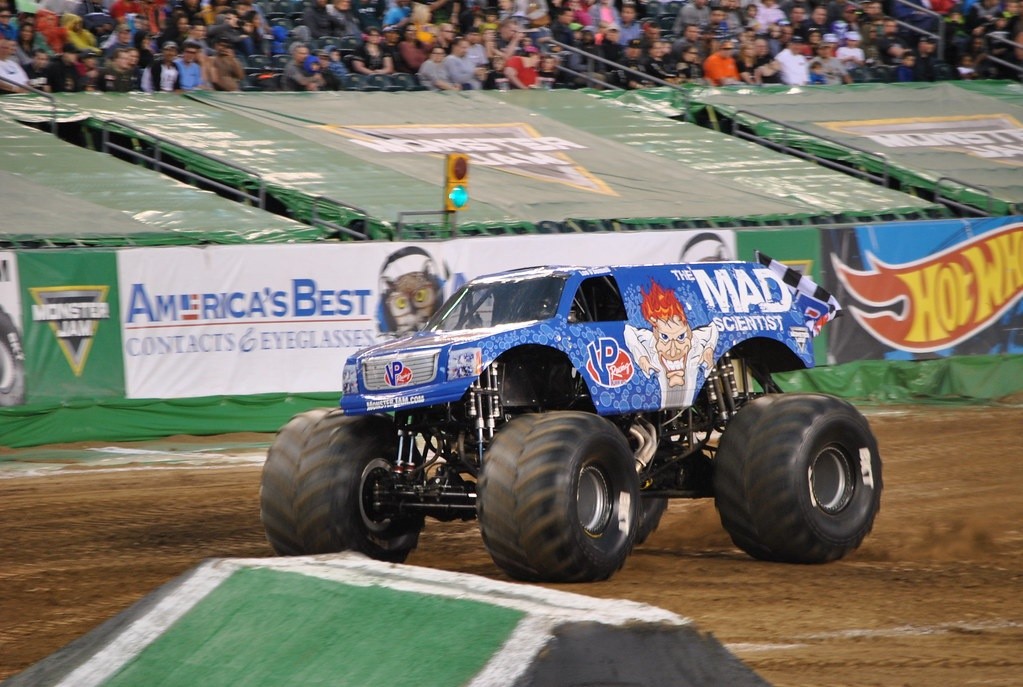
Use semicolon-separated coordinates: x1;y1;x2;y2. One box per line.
260;252;884;583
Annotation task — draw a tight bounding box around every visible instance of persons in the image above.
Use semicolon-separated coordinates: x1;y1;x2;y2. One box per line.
0;0;1022;97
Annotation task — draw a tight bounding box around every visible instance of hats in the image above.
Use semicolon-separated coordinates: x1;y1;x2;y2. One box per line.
628;38;646;49
778;20;791;27
183;39;203;49
843;30;861;41
162;40;180;50
116;23;131;32
523;45;538;53
305;55;321;74
581;25;596;34
823;33;840;43
568;23;584;31
790;36;804;43
605;24;622;32
76;49;102;58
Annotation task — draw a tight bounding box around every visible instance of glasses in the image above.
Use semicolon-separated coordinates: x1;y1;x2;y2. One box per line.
184;50;197;54
445;30;457;33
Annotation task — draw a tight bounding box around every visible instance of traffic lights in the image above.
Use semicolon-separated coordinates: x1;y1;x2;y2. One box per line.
443;150;470;211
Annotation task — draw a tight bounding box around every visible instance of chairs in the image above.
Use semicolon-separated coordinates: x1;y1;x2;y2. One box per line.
83;0;684;92
